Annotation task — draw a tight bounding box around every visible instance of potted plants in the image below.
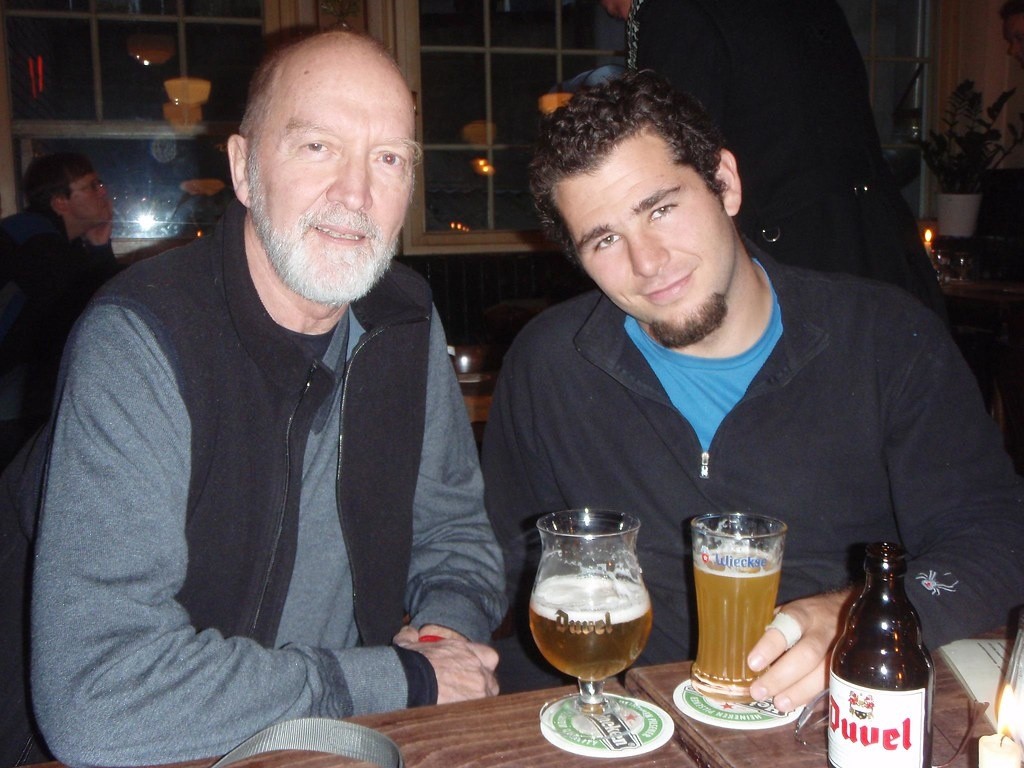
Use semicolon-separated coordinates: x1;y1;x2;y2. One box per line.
907;79;1024;242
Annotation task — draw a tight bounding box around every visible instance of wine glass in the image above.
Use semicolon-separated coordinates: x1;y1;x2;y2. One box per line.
530;510;651;738
933;249;975;287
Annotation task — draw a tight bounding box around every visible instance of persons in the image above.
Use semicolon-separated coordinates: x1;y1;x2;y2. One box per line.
0;150;124;467
604;0;949;331
0;31;506;768
481;72;1024;712
1000;0;1024;70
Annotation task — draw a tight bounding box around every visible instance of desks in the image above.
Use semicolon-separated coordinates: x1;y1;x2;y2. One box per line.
20;619;1024;768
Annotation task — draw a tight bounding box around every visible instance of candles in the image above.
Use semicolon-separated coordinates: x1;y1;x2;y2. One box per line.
924;229;933;258
978;683;1023;768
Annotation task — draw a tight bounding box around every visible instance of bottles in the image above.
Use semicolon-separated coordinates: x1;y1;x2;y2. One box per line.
828;543;935;768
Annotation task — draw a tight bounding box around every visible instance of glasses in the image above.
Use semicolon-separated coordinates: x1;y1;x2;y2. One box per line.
65;180;104;195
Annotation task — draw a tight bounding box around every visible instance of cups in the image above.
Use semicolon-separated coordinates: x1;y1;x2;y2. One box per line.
692;512;788;701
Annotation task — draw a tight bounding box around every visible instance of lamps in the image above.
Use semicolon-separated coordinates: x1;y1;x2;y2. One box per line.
458;90;574;147
124;33;227;198
